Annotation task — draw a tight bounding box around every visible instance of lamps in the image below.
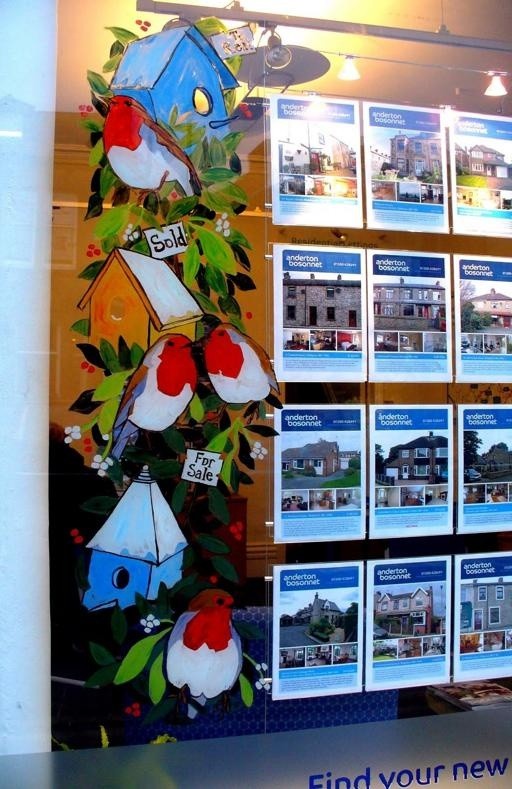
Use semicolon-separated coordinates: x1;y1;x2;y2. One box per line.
260;18;293;72
